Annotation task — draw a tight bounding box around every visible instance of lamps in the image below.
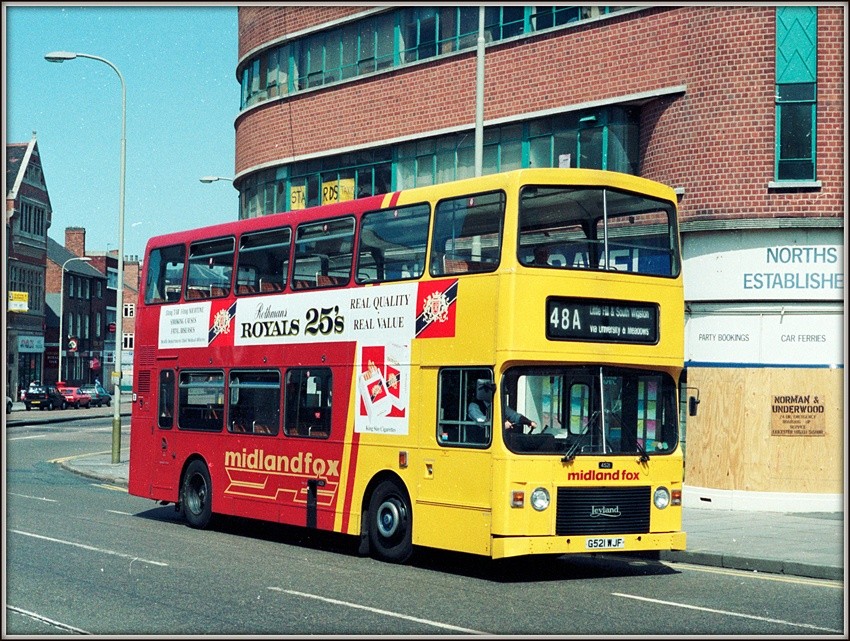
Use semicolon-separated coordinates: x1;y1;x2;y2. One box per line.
199;176;235;184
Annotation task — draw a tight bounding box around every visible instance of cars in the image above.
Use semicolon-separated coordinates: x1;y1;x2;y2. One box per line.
6;395;13;413
57;387;92;410
79;384;111;407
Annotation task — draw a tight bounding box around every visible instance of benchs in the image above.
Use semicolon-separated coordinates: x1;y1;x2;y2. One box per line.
253;419;279;434
308;424;331;437
154;253;509;303
232;420;252;433
285;424;307;435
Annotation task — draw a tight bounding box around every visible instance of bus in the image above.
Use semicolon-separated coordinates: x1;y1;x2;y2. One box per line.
127;165;701;563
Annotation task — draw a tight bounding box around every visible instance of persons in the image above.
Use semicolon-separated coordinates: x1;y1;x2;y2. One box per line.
468;382;538;443
30;379;40;386
524;244;553;267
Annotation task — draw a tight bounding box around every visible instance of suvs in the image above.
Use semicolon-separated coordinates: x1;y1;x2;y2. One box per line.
24;384;68;410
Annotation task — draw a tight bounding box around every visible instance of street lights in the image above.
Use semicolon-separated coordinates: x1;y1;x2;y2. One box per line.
57;255;91;382
42;49;123;467
